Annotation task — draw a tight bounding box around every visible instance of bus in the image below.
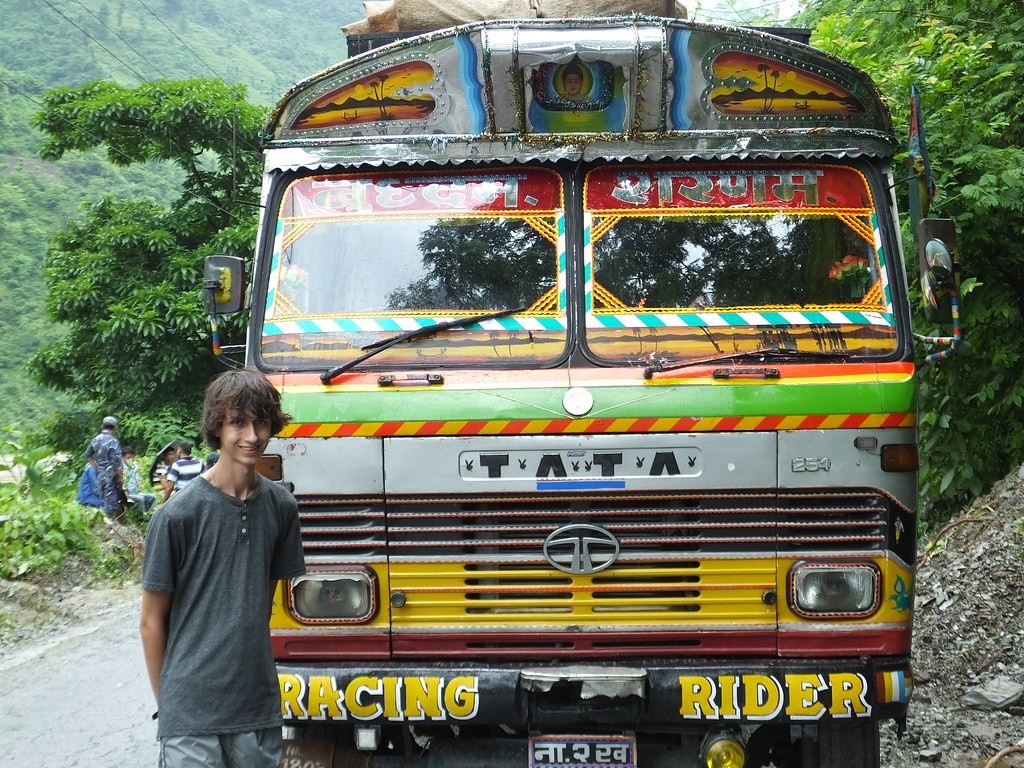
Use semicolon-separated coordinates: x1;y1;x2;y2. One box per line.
201;11;965;767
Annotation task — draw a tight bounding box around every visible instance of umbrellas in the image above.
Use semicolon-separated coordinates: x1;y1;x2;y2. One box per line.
147;440;176;480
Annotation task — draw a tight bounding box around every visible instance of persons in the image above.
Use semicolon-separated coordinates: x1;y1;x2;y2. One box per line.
163;443;203;502
122;446;155;516
198;452;219;477
153;447;175;489
77;456;100;507
86;416;127;528
140;370;306;768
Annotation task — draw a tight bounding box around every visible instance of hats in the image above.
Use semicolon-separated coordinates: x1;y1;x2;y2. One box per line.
206;453;219;466
103;416;117;428
